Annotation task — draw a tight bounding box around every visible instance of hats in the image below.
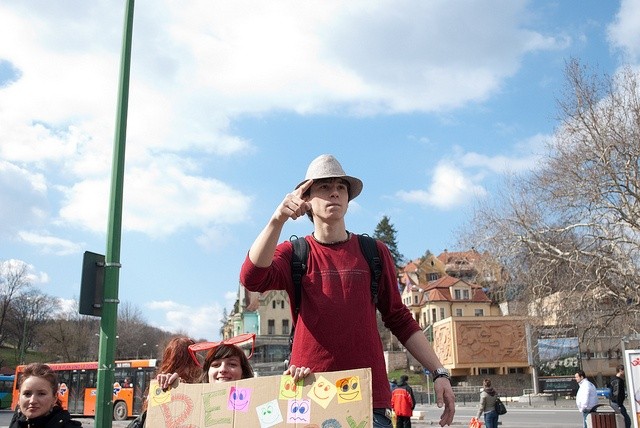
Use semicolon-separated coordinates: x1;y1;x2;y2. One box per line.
295;154;363;200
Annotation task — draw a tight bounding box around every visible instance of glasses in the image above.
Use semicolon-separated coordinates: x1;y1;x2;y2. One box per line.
188;333;255;369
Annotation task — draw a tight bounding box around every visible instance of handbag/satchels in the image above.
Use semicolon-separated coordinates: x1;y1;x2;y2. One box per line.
495;392;507;415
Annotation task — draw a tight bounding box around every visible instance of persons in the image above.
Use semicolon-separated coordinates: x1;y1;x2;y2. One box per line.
475;378;498;428
238;153;455;428
8;363;84;428
574;370;598;428
609;364;630;428
142;337;207;411
390;375;416;428
389;377;397;391
156;344;313;390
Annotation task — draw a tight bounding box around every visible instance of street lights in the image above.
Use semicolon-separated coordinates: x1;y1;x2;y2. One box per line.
412;285;430;405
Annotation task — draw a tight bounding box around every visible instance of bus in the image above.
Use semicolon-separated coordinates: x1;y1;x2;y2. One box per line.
12;359;171;420
0;375;17;411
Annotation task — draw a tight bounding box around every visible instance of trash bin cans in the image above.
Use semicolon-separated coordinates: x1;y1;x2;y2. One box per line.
586;403;626;428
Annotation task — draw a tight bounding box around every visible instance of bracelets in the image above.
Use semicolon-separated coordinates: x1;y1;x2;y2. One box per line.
431;367;451;382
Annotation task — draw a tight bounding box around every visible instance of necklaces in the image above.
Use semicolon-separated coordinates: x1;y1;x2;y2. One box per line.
311;230;351;245
